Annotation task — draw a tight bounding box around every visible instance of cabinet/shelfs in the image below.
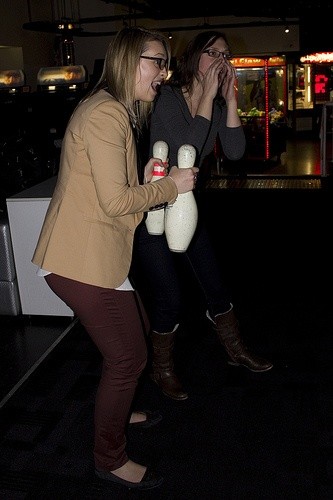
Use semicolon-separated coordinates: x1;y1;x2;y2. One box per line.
5;176;75;320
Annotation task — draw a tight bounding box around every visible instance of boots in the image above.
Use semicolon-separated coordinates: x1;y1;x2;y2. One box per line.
206;302;273;372
150;323;188;400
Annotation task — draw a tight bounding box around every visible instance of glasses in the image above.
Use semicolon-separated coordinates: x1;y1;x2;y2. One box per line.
203;49;232;61
141;56;169;71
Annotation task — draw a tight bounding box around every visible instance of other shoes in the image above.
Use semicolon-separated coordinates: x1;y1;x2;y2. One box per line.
95;464;162;490
128;408;166;428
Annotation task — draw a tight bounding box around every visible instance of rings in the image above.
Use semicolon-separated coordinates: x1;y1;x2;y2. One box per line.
194;176;196;180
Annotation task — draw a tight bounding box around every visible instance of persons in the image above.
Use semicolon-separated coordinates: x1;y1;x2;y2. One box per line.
250;81;258;105
133;31;274;401
31;26;199;492
